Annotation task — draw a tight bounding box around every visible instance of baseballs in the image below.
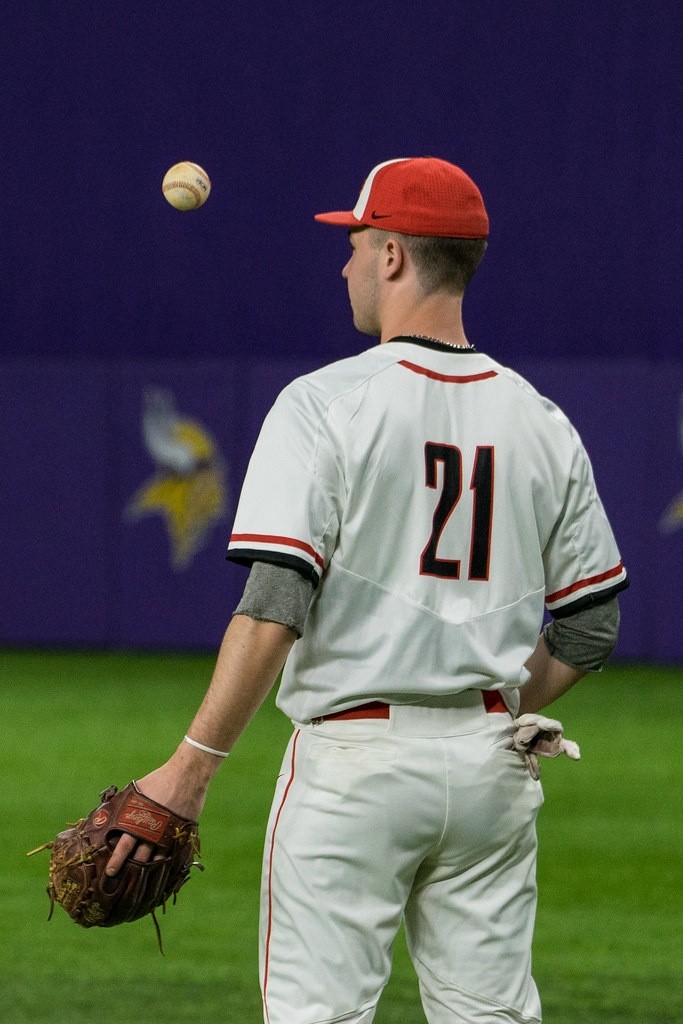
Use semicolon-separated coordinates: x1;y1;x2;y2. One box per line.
160;160;212;211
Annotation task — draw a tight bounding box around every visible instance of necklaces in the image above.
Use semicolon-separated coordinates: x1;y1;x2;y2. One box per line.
402;334;476;350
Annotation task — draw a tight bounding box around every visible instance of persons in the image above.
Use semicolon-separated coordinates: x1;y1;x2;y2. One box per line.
26;157;632;1024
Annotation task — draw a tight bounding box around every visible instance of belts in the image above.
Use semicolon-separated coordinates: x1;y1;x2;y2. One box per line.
311;690;508;717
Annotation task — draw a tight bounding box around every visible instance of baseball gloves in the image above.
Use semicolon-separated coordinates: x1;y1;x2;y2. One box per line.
47;778;204;928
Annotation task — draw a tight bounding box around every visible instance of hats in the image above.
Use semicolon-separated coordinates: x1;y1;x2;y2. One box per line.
313;158;490;238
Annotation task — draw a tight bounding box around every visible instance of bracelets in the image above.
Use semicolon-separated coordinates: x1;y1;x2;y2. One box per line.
181;735;230;758
